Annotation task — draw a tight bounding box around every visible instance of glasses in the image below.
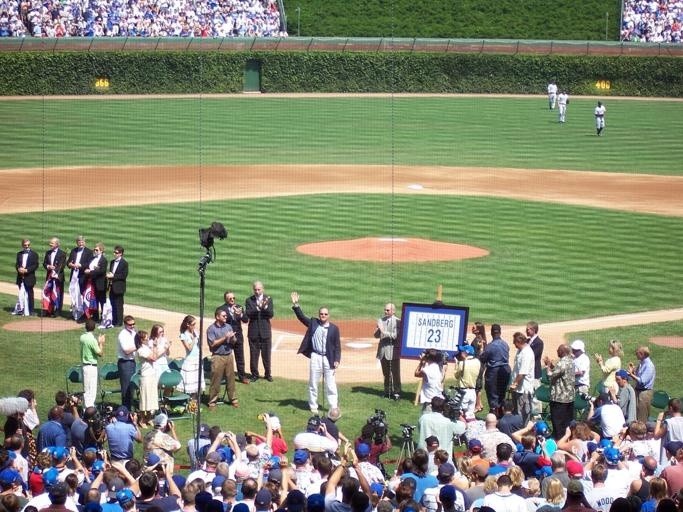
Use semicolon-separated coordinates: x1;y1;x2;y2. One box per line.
230;297;235;300
129;323;135;326
113;251;120;254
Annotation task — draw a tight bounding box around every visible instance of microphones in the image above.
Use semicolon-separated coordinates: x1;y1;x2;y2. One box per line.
320;327;328;339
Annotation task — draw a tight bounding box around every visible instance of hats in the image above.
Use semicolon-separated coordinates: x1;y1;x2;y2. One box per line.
587;439;620;465
200;416;280;448
207;451;282;511
535;420;548;434
571;340;586;352
0;445;106;491
663;441;681;457
615;369;632;382
109;406;169;503
458;344;474;355
536;460;584;495
425;435;482;503
308;416;320;427
492;324;501;333
289;443;384;510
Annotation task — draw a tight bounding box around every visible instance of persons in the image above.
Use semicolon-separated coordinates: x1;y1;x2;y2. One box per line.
1;281;682;510
595;101;606;136
0;0;289;38
83;243;108;322
558;90;569;123
67;236;92;321
43;237;67;318
547;80;558;109
620;0;683;44
15;239;39;316
105;246;128;328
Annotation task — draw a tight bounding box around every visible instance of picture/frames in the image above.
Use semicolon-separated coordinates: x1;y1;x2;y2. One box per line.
397;302;469;363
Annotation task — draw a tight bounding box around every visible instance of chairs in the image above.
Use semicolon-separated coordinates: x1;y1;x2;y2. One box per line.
64;357;195;424
526;366;672;439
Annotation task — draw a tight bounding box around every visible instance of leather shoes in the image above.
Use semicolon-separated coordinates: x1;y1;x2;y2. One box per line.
208;405;216;412
250;376;258;382
265;376;273;382
232;403;240;408
241;376;249;384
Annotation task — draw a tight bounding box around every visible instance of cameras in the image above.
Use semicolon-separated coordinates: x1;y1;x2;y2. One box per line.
400;423;416;439
224;434;229;438
105;463;112;468
155;464;167;471
165;419;172;431
63;447;71;454
128;413;138;424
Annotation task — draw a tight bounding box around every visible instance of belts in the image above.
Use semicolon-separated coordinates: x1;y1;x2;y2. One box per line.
313;351;327;355
83;363;97;366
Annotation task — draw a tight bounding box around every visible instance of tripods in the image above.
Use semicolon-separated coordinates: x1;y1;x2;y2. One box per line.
393;441;417;475
